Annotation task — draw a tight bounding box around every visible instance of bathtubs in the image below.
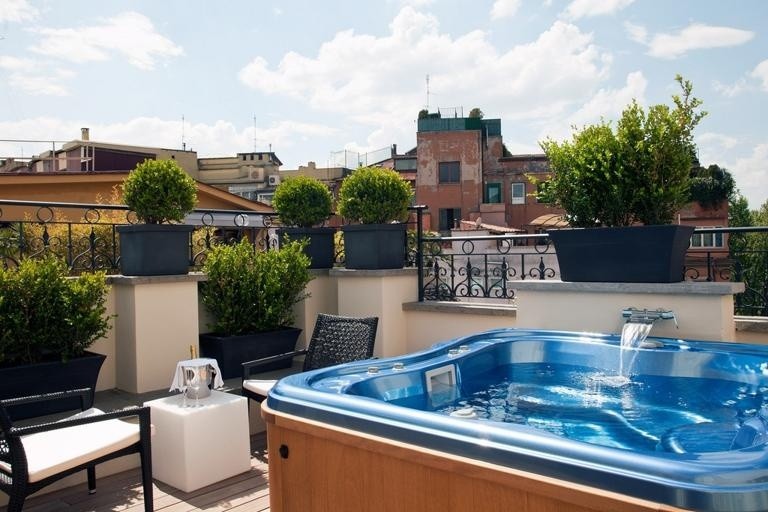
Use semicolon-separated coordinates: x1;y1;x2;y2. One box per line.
260;325;768;511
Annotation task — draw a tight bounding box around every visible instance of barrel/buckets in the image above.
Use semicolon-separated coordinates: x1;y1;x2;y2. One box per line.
182;364;215;400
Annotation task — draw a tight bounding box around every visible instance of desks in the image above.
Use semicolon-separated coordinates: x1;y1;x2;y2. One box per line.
143;388;251;493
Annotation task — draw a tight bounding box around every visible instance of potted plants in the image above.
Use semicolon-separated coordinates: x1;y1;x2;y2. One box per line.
197;236;312;374
530;72;709;282
0;254;116;423
115;157;414;275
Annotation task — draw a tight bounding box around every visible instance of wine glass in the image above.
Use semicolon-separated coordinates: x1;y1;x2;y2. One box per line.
178;366;204;409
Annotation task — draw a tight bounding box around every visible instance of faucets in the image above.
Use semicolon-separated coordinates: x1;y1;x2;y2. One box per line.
620;308;673;327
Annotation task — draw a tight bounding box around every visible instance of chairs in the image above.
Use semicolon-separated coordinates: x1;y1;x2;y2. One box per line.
241;313;378;436
0;387;154;512
